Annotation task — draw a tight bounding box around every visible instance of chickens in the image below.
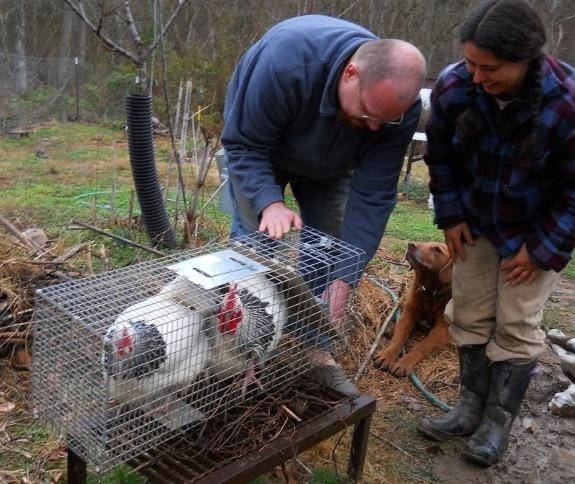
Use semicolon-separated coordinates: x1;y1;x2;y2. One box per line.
203;274;287;397
100;275;219;416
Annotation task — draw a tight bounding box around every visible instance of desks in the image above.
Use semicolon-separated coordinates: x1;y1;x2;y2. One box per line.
66;364;375;479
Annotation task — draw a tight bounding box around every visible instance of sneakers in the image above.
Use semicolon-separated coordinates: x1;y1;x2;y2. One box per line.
307;364;361;398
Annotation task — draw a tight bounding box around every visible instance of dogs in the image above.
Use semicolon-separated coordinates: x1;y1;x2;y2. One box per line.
373;240;453;377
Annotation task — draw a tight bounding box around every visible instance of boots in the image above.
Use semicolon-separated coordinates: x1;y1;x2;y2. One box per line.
415;344;490;443
461;359;538;466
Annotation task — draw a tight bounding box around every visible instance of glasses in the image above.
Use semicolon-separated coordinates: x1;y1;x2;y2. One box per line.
357;95;404;127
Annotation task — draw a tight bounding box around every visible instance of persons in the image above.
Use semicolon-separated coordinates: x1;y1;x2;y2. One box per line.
425;0;575;468
221;16;426;396
